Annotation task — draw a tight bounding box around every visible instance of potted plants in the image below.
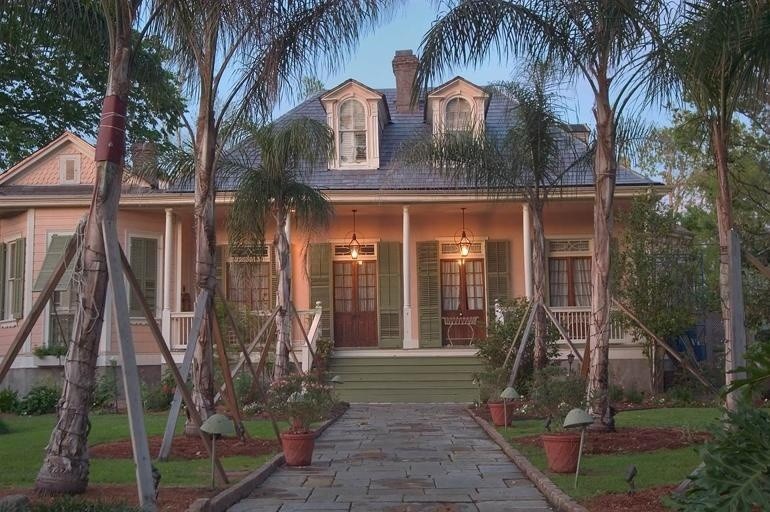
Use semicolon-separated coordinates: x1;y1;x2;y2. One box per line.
523;366;603;473
267;370;337;467
486;368;518;426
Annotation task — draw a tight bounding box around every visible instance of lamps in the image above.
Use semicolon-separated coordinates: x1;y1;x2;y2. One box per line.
348;209;361;259
458;207;472;257
